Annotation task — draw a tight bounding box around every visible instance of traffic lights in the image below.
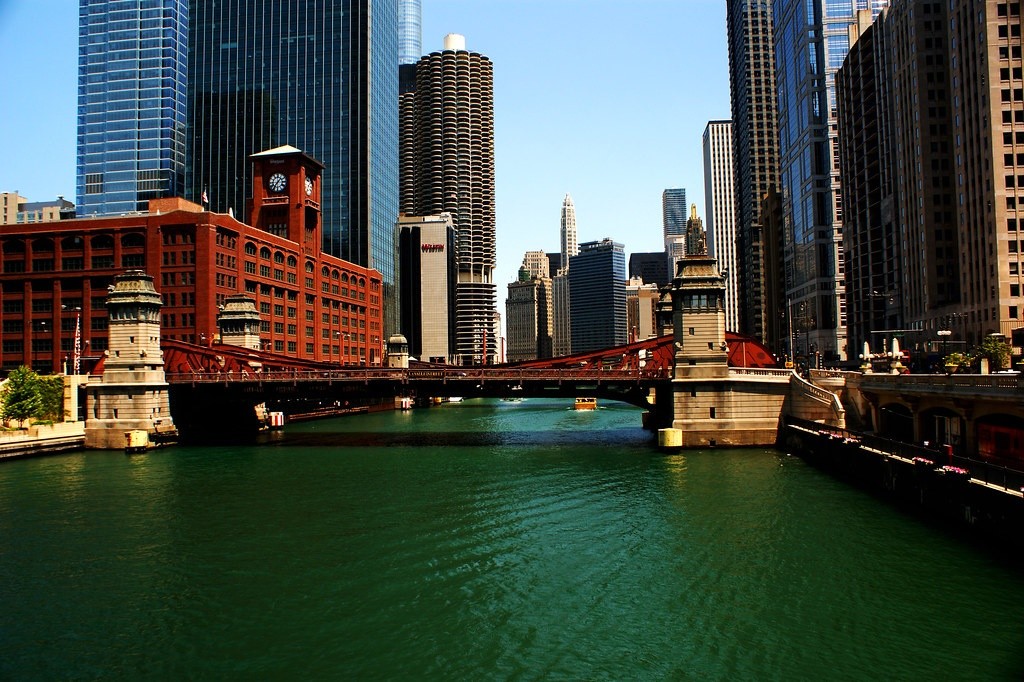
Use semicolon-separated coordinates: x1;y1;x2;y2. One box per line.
928;338;931;351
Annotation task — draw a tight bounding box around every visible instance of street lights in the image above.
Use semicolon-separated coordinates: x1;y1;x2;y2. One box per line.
800;297;810;368
375;339;386;367
781;299;793;361
61;305;81;375
938;330;951;372
29;322;45;372
336;332;350;366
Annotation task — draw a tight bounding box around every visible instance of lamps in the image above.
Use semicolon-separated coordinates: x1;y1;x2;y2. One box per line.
719;340;727;351
675;342;682;350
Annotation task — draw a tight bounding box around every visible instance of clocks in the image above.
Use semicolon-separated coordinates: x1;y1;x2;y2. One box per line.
268;172;288;193
304;176;313;196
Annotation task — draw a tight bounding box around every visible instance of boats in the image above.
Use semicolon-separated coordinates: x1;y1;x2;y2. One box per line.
574;398;597;410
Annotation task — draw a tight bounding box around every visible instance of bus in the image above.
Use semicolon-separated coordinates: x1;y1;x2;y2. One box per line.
870;351;910;374
